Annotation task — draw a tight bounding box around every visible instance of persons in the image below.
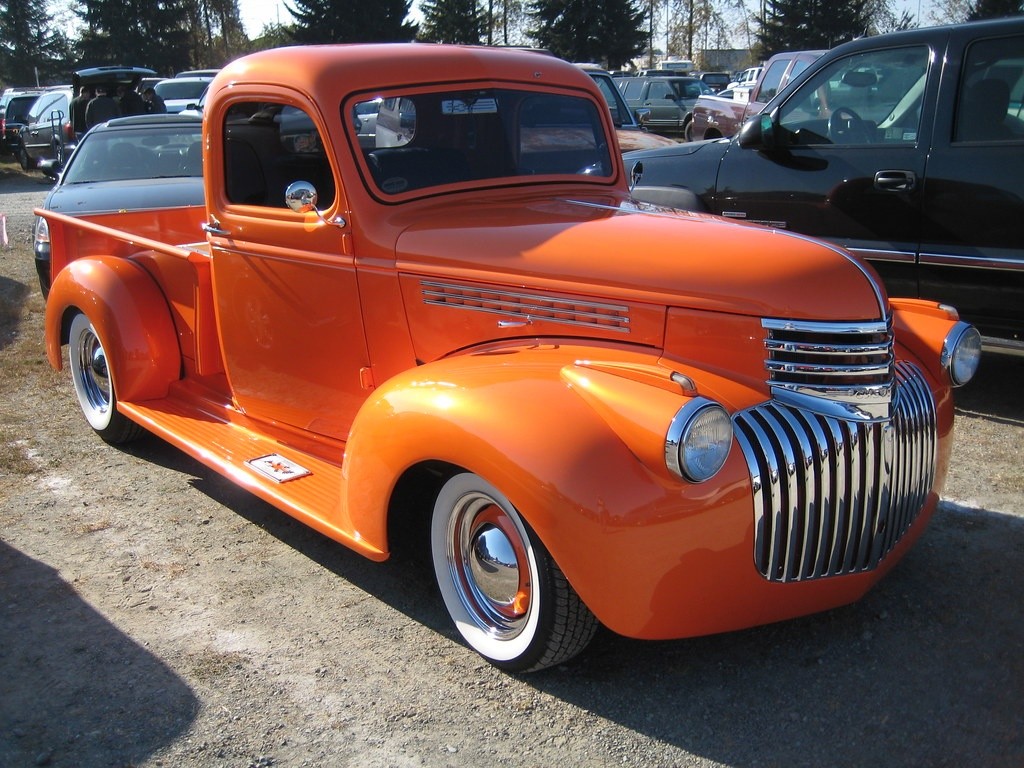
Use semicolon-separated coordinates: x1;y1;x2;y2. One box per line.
85;85;121;132
145;87;167;114
116;84;147;117
68;85;92;143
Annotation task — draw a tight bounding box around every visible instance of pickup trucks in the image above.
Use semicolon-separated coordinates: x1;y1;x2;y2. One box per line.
33;42;984;675
691;50;908;143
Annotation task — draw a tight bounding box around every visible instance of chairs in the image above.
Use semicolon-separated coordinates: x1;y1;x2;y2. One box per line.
952;78;1016;142
369;146;451;190
182;141;203;177
99;143;147;179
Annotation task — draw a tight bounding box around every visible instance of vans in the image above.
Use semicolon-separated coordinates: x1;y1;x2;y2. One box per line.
597;76;718;141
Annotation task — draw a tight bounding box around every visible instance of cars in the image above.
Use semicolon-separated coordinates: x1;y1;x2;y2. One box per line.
0;85;73;156
685;86;756;141
17;65;159;182
690;71;732;95
353;98;383;135
633;68;677;77
272;99;318;133
376;63;681;180
621;16;1024;399
152;77;213;114
173;69;220;79
608;70;635;76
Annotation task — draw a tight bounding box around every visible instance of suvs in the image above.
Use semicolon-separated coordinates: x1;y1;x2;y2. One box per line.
726;67;764;89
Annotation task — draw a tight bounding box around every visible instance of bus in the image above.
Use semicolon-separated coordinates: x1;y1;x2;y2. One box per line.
656;60;694;74
